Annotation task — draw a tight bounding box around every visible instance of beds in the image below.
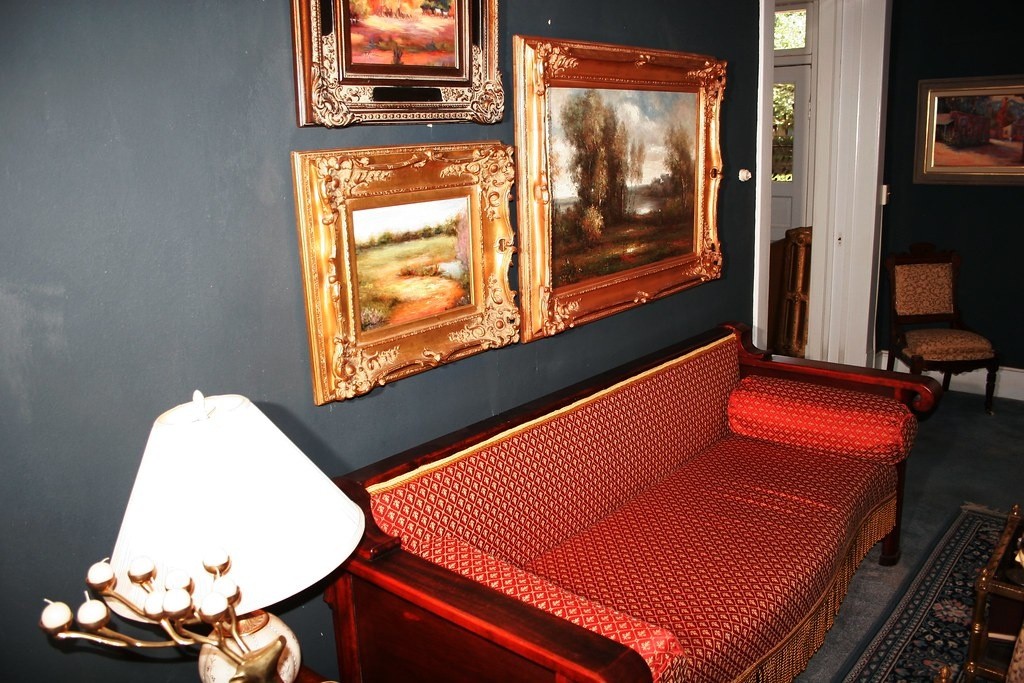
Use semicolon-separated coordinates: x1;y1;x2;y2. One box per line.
768;225;813;358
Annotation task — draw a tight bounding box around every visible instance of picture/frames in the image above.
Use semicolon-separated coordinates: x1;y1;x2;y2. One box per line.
912;75;1024;187
291;138;522;405
510;35;729;344
291;0;505;129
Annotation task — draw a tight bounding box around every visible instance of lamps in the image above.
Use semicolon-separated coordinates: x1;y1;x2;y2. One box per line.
38;390;365;683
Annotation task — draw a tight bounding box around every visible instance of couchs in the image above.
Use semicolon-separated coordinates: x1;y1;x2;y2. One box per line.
317;323;942;683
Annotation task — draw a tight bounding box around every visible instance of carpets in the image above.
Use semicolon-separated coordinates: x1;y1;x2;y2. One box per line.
830;500;1008;683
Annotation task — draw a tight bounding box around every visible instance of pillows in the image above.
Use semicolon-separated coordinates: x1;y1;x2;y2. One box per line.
726;374;920;467
410;535;693;680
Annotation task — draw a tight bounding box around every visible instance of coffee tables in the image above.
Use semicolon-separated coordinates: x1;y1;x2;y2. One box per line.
961;504;1024;683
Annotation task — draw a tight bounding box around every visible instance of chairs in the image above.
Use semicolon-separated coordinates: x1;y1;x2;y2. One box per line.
886;241;1001;416
